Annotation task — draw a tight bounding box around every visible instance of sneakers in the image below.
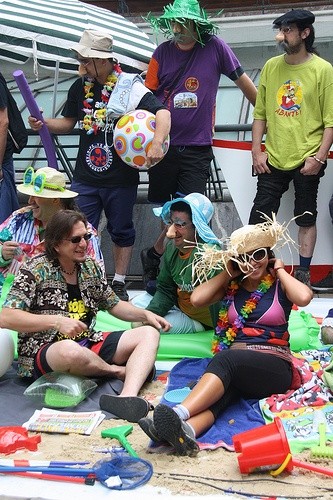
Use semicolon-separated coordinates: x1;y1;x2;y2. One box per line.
138;417;162;443
139;247;160;296
110;281;129;301
311;272;333;293
292;268;311;288
153;403;201;456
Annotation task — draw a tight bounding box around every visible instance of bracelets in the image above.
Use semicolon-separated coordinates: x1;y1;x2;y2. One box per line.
313;156;325;164
273;266;283;273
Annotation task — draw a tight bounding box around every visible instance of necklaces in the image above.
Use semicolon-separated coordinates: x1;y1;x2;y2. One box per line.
211;273;277;354
56;263;78;275
82;63;122;134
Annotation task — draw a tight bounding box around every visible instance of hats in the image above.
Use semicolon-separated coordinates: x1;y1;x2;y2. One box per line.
191;211;298;274
159;192;221;243
144;0;224;47
273;8;315;25
16;167;79;199
70;29;115;59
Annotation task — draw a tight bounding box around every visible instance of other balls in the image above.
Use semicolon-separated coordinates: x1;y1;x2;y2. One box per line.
114;110;172;168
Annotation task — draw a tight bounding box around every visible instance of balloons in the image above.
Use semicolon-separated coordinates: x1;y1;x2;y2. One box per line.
113;110;170;170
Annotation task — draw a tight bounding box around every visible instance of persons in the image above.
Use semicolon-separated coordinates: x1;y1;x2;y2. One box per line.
130;192;232;334
310;193;333;291
141;17;259;296
0;73;20;224
139;247;313;456
0;210;172;423
0;166;106;356
249;9;333;285
318;308;333;344
29;30;171;300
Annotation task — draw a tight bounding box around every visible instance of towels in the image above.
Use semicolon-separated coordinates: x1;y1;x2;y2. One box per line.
108;73;153;114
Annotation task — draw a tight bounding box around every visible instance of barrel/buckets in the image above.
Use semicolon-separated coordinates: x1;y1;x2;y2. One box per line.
232;417;293;477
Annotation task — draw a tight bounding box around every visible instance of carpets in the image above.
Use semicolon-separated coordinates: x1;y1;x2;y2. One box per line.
1;298;333;500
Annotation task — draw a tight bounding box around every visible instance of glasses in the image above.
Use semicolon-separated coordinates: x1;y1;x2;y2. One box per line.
167;217;192;228
272;26;296;34
76;58;90;66
234;248;269;263
62;232;92;243
23;167;66;194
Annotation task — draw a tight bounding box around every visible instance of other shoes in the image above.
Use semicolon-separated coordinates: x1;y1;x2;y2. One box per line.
146;366;157;382
100;394;148;423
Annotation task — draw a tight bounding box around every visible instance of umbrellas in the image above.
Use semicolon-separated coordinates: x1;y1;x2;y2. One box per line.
0;0;158;119
